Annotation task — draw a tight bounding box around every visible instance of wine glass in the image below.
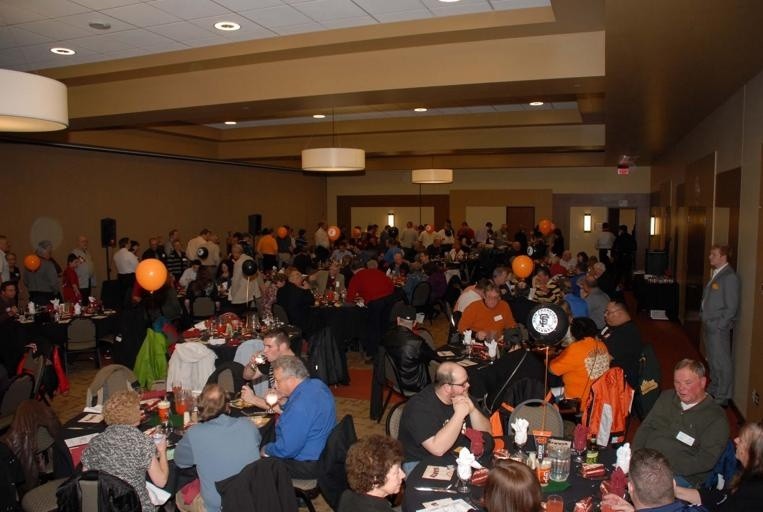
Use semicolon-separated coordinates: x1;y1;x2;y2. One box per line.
486;347;496;366
152;433;167;452
17;299;102;320
250;352;267;370
465;341;475;359
266;389;278;414
514;429;527;458
205;313;285;337
601;496;619;512
457;464;471;494
547;495;563;512
171;381;182;403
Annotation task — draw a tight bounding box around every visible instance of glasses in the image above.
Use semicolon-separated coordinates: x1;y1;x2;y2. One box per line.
448;378;470;387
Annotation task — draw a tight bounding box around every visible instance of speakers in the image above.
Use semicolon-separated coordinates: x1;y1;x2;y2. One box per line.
248;214;262;236
100;218;116;248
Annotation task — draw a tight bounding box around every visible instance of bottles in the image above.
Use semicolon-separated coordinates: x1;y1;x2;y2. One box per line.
586;434;599;467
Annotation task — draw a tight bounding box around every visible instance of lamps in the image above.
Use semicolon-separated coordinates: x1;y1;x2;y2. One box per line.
387;213;395;227
0;67;70;134
301;104;367;173
583;212;592;233
411;153;454;185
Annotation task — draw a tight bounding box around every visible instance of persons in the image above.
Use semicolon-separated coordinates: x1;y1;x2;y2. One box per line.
330;223;762;512
1;216;353;512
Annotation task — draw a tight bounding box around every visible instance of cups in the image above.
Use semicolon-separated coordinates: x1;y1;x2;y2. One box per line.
537;461;550;485
550;446;571;480
158;401;172;421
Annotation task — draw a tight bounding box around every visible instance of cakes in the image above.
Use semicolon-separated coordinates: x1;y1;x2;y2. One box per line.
573;496;593;512
471;468;490;485
493;448;510;460
580;463;606;478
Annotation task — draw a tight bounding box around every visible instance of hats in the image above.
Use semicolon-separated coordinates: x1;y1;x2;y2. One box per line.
68;252;78;263
398;305;416;320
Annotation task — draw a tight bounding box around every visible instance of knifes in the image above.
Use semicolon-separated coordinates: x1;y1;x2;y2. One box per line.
413;485;459;496
